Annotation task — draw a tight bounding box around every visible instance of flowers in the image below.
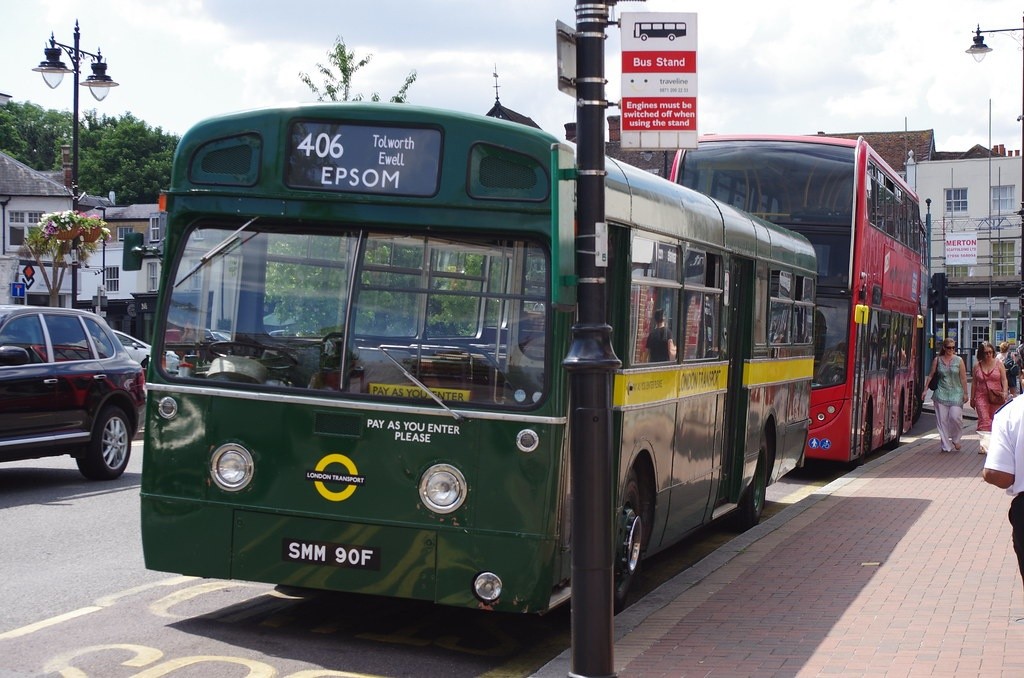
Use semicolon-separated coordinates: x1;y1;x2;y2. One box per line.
25;210;112;261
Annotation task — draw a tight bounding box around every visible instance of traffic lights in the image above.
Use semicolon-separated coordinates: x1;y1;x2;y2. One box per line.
927;287;939;310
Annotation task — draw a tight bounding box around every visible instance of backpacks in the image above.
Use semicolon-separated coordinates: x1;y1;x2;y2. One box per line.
1003;352;1019;377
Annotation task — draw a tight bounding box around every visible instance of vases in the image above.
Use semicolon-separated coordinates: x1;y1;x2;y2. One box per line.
54;223;81;240
81;227;101;242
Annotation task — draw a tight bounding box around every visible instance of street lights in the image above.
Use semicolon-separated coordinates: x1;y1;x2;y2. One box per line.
33;34;121;304
990;297;1007;341
94;206;106;296
964;27;1024;392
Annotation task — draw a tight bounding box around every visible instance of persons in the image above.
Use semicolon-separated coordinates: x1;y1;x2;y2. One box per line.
922;338;969;451
997;342;1024;398
179;323;200;364
970;341;1008;453
983;363;1024;589
642;310;676;362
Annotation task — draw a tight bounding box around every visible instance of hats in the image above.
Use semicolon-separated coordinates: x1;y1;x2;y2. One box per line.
654;309;664;319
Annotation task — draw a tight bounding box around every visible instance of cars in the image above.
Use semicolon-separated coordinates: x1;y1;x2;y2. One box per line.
114;329;180;376
202;327;314;342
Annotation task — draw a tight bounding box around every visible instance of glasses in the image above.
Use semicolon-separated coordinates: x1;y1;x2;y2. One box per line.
984;351;993;355
944;346;956;349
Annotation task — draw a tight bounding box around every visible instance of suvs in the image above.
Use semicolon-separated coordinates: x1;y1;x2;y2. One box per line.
1;306;145;480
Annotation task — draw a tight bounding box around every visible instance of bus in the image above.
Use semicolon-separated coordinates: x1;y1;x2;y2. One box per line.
668;131;923;461
121;104;819;614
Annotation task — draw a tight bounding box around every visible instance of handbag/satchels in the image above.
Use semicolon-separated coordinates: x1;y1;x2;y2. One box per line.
987;389;1005;404
928;357;941;390
